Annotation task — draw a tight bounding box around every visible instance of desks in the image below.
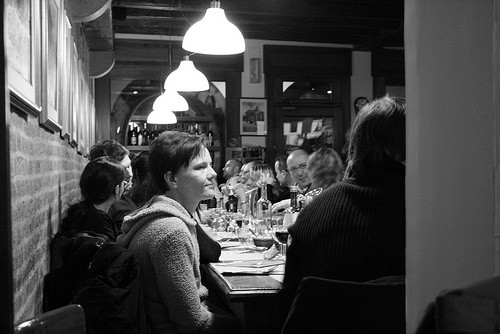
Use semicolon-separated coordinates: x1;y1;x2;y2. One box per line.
197;224;284;334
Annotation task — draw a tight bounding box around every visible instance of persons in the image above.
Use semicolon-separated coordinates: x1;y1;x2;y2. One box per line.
115;130;238;334
219;145;347;216
57;139;157;269
275;95;405;334
247;105;261;124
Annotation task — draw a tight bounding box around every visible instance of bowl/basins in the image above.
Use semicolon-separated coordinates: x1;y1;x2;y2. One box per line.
252;239;274;250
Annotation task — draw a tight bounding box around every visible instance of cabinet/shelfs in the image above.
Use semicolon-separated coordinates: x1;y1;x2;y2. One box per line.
125;92;221;183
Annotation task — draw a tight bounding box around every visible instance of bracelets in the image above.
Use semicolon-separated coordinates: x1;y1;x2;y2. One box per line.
298;194;306;209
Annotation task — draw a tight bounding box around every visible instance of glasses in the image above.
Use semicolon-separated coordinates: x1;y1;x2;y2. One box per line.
288;163;307;173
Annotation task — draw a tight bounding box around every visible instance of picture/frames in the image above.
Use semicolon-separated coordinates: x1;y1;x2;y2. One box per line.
4;0;95;160
239;97;268;136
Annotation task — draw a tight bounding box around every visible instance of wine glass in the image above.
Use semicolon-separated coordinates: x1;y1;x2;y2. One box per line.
209;210;251;245
271;225;290;261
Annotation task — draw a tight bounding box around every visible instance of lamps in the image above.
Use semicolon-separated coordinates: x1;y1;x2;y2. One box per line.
147;40;210;124
182;0;245;55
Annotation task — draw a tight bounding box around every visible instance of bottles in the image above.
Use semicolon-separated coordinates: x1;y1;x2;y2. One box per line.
256;182;272;221
145;125;165;145
194;130;213;146
225;195;237;213
130;129;143;146
289;186;300;224
242;145;265;158
